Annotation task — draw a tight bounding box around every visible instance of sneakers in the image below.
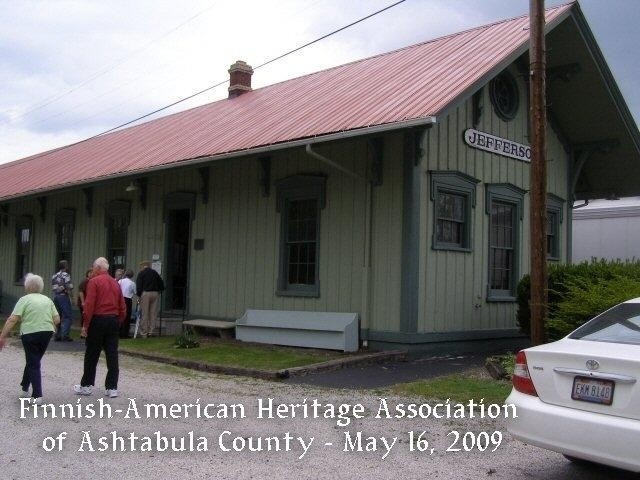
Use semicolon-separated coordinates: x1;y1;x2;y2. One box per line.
73;385;95;396
18;388;29;398
27;397;43;406
104;388;119;398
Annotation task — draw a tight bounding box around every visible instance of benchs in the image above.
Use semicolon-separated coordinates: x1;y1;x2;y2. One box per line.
182;318;236;341
235;309;359;352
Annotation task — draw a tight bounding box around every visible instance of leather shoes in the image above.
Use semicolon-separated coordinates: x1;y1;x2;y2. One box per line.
54;336;61;340
62;336;74;341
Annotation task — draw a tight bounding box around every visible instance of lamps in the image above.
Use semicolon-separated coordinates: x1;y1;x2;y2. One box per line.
124;181;162;193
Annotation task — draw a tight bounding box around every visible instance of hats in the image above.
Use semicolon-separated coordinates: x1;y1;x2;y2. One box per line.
140;261;149;266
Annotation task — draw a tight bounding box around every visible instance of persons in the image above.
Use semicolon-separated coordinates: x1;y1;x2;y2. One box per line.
117;269;138;339
136;260;165;336
0;272;61;406
74;257;128;398
52;260;73;342
113;268;125;281
77;268;92;339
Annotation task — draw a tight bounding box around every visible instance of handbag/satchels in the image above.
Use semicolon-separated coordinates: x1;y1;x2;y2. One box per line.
131;311;141;323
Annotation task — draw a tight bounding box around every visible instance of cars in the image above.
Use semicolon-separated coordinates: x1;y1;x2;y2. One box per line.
501;296;640;473
